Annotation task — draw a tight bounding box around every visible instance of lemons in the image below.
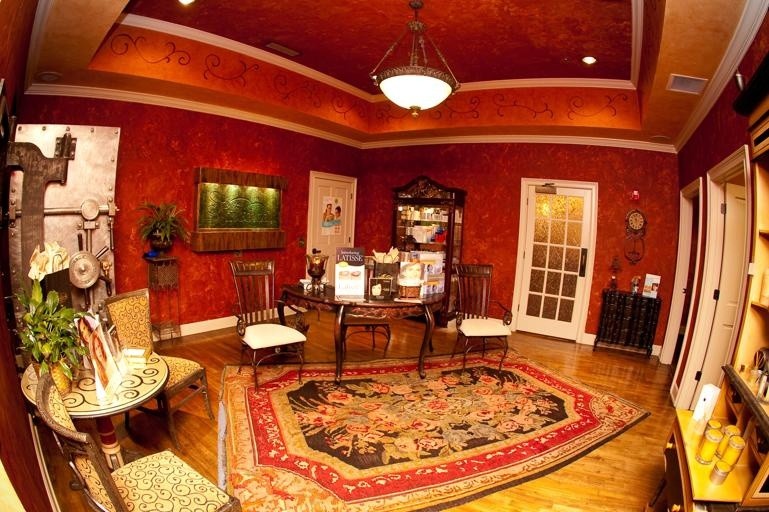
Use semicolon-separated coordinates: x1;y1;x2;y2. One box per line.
754;371;768;403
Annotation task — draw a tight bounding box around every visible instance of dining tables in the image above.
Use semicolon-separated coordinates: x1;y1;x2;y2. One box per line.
135;201;192;253
12;281;99;399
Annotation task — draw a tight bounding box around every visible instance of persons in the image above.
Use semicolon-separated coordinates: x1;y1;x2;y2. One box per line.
426;286;431;296
77;315;95;370
653;283;658;291
432;285;437;294
333;206;341;225
88;331;115;391
398;262;421;287
427;263;433;275
322;204;334;225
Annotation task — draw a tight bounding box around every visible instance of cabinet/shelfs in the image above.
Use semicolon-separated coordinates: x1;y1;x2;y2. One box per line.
631;278;641;295
610;275;617;290
694;418;746;487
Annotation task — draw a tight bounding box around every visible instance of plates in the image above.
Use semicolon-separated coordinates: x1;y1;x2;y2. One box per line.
592;288;661;357
395;178;466;327
724;143;769;508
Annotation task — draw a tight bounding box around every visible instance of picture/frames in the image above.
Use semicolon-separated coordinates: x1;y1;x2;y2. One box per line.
147;254;180;346
279;284;450;380
19;347;167;487
648;410;761;512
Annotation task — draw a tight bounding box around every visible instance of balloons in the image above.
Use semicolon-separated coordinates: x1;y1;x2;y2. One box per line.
623;208;648;264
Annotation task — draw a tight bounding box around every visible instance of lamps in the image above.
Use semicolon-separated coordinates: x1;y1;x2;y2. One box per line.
229;260;308;392
34;372;239;512
451;263;512;371
105;288;216;451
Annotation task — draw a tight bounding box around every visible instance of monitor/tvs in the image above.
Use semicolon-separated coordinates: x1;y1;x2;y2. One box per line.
642;273;661;299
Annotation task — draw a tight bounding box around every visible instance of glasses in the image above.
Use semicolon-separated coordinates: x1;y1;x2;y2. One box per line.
368;0;457;118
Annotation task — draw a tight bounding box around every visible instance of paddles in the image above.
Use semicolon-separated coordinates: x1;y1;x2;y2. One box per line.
217;346;649;512
